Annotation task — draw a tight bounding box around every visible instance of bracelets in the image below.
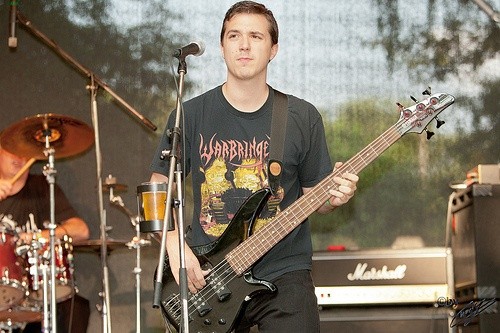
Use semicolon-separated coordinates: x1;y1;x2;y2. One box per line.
326;199;338;209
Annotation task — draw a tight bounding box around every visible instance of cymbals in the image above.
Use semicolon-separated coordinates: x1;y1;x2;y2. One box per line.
70;239;129;251
0;114;94;160
95;184;128;193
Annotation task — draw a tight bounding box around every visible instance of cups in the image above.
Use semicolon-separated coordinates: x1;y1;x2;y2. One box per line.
140;181;166;220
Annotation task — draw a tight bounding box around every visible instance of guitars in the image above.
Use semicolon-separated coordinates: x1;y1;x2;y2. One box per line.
153;86;454;333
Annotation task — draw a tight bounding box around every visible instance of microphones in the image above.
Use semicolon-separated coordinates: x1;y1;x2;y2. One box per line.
173;39;204;58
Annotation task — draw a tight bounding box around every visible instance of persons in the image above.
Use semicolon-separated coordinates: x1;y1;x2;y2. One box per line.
0;147;91;333
149;0;359;333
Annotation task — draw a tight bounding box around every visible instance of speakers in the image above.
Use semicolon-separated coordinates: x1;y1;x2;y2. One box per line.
319;305;464;332
450;184;500;333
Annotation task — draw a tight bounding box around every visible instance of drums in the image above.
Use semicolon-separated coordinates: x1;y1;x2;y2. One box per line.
0;228;24;313
25;237;75;304
0;273;50;322
